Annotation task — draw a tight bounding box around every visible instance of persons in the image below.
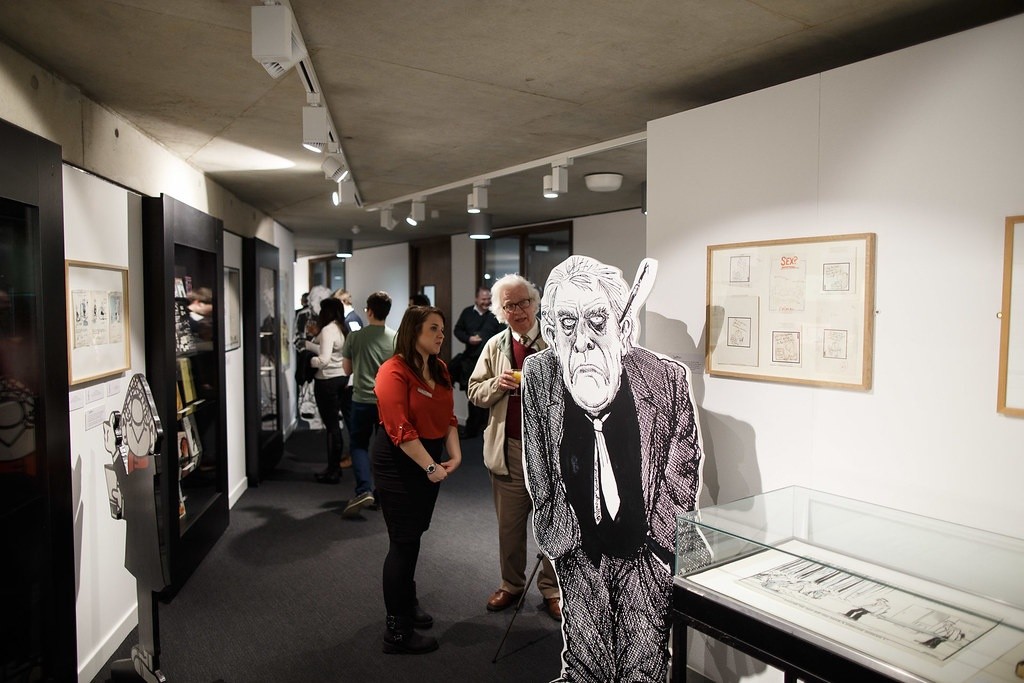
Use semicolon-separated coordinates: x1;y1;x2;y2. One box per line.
368;306;462;655
341;292;395;517
408;293;430;310
467;272;563;621
300;298;352;483
297;291;317;382
338;293;364;332
453;287;508;454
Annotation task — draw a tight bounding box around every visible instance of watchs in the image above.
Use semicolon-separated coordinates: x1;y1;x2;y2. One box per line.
426;462;436;474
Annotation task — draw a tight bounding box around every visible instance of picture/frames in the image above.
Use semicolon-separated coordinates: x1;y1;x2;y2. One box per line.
223;266;241;354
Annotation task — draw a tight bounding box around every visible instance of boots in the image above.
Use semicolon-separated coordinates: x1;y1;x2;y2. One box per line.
314;433;344;484
410;581;433;629
382;615;438;654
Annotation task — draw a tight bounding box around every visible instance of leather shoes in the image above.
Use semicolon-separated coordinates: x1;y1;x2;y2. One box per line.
542;596;563;621
487;589;524;611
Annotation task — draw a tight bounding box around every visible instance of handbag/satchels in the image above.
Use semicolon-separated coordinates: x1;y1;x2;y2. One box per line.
447;353;465;382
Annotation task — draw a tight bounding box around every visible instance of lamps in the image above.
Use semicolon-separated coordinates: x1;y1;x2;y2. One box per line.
406;202;425;227
332;181;354;206
379;210;398;231
251;3;307;80
467;183;489;214
543;168;568;198
469;210;491;240
336;240;353;258
322;156;348;183
301;93;330;153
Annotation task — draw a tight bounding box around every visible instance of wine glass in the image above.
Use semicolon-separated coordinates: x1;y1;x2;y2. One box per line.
510;370;521;396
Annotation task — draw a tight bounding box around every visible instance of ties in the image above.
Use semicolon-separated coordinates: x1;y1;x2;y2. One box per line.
521;337;528;345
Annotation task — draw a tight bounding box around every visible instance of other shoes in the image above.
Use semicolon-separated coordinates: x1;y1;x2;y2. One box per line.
344;490;374;518
458;430;477;440
376;504;383;512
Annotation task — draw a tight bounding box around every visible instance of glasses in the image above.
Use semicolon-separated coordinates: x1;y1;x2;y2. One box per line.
363;306;369;312
502;298;530;313
